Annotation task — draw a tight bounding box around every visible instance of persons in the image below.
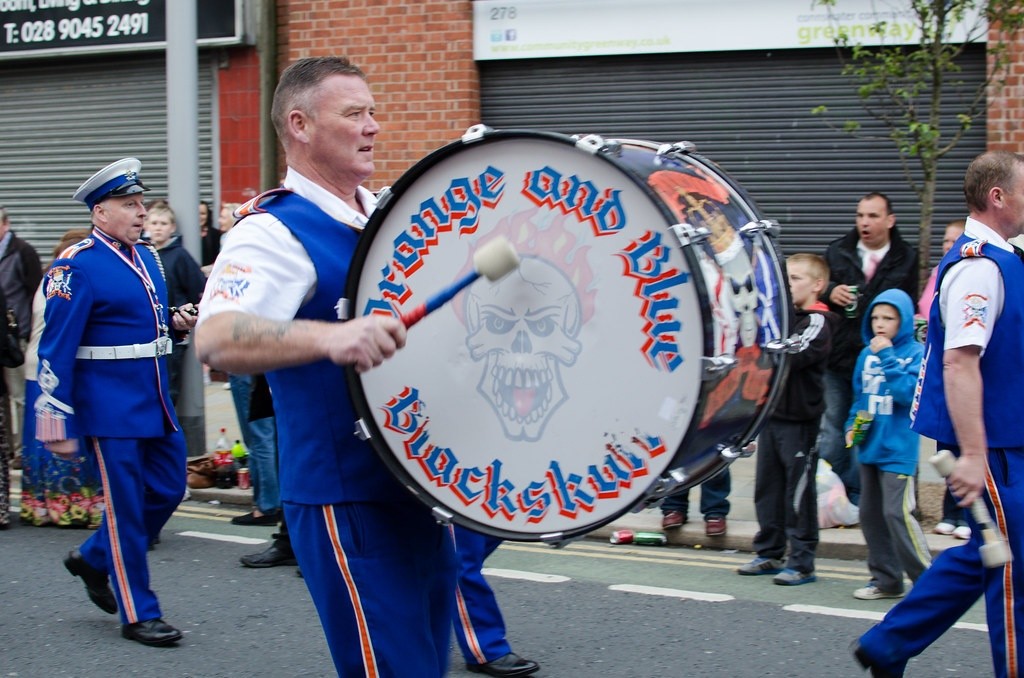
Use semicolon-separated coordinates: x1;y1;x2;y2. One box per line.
192;53;463;678
32;157;187;644
850;149;1024;678
0;189;968;603
446;525;539;676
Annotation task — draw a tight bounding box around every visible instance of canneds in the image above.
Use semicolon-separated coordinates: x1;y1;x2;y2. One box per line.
844;286;859;318
610;530;635;544
913;319;928;345
633;532;667;546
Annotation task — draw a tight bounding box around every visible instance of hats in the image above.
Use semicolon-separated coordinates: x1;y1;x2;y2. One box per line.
73;158;151;210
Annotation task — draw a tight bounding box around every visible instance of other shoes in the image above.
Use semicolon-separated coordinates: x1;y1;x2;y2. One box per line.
0;523;7;531
13;456;21;469
233;511;282;526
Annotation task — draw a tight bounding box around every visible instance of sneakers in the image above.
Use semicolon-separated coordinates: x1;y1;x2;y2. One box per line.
854;584;904;600
933;522;956;534
951;526;971;539
773;569;815;585
706;516;725;535
663;511;684;529
737;557;783;575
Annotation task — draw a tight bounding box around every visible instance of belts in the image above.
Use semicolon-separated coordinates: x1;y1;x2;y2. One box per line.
76;337;172;359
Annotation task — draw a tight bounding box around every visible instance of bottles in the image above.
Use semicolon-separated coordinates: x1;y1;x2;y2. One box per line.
213;428;236;489
231;439;246;486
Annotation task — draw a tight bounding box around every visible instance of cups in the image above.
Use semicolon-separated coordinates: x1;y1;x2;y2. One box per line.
237;467;250;490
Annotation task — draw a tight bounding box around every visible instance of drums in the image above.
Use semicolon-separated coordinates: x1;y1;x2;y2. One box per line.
337;122;797;545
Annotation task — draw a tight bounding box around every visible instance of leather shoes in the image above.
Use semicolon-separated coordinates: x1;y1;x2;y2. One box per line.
122;619;182;645
850;640;903;678
240;547;298;567
63;546;117;615
